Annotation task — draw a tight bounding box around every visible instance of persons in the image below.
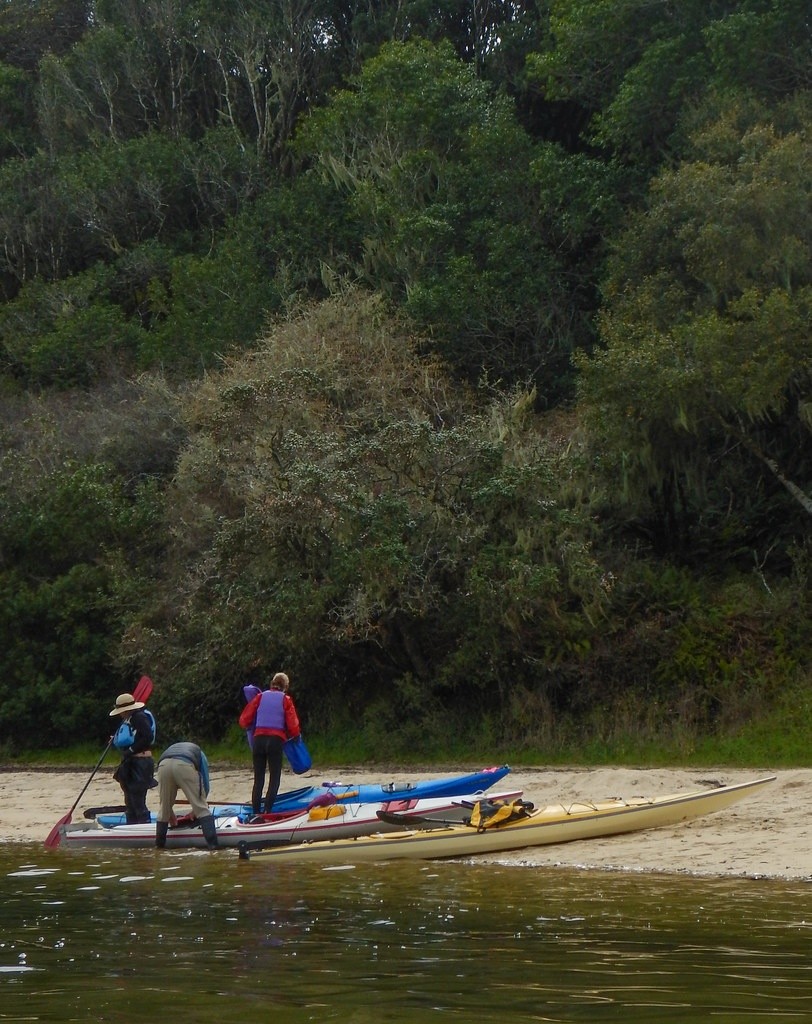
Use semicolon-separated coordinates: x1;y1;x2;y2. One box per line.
154;740;227;851
108;693;159;825
238;672;301;817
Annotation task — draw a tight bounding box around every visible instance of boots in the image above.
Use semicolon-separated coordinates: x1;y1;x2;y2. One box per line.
155;821;169;848
200;815;221;850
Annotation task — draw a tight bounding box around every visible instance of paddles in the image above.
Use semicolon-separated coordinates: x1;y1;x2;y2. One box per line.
374;809;471;827
43;674;155;849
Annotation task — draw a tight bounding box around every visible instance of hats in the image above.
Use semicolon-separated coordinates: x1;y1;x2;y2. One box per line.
109;694;145;716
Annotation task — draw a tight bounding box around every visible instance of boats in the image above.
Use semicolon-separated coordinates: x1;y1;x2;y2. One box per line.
235;775;779;864
59;789;526;850
96;764;511;831
